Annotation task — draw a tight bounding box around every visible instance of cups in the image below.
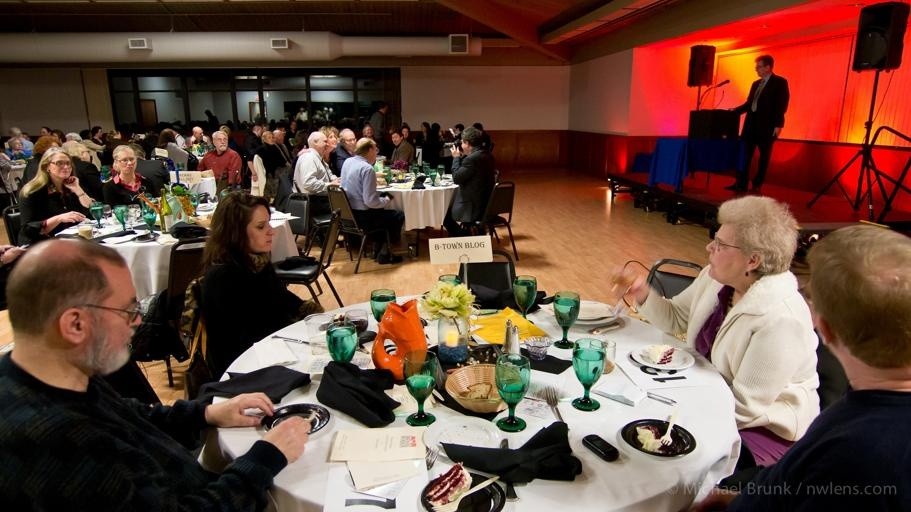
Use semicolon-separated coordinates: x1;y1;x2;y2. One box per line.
524;335;551;362
304;314;332;355
604;340;616;375
77;224;94;239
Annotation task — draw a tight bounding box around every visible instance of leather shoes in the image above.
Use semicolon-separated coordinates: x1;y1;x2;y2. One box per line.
723;182;762;192
373;249;403;264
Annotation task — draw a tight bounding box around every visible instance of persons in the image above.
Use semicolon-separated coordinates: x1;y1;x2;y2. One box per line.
193;187;325;381
0;99;498;313
610;195;821;481
724;54;789;191
683;223;911;512
0;237;310;510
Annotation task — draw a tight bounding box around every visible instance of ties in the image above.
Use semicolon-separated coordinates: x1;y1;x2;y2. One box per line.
751;79;766;112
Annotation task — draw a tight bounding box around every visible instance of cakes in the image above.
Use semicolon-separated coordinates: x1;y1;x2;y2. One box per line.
425;462;473;506
636;425;665;454
649;344;674;365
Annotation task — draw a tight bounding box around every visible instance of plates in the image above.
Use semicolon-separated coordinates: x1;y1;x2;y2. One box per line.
421;415;501;456
261;403;330;436
620;418;696;459
420;472;506;511
548;299;620;326
630;342;695;371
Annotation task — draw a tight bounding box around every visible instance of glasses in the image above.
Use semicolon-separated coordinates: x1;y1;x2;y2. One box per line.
714;232;741;250
85;300;141;323
116;157;136;163
50;160;71;166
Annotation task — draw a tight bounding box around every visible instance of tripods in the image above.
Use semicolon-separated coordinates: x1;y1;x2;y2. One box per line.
805;72;892;221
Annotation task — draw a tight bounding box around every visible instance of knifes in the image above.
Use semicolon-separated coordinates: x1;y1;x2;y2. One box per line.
500;437;524;503
273;335;328;351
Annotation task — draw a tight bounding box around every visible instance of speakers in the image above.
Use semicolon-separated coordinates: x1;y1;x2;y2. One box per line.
852;2;911;71
687;44;716;88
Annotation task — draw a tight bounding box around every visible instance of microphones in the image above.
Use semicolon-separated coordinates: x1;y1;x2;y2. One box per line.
697;80;730;110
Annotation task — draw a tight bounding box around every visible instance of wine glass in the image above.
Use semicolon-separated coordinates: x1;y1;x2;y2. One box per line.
551;289;581;350
382;161;445;187
569;337;606;413
326;321;359;364
370;288;398;322
343;309;369;334
176;161;184;170
495;353;530;433
402;349;440;427
437;274;461;285
513;274;538;325
87;187;228;236
184;144;215;157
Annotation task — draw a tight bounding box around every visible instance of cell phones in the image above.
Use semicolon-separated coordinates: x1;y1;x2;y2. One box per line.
582;434;620;462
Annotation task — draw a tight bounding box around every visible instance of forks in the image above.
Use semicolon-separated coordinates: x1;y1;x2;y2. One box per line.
425;446;441;469
432;476;500;512
544;386;570;432
659;409;680;447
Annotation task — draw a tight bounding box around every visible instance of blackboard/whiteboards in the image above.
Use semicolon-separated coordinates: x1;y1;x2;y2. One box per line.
712;32;911;150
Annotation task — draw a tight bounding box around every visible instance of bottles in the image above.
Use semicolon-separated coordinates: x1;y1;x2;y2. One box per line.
500;318;522;361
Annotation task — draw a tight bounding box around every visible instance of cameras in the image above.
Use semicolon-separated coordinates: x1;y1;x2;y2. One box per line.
454;139;462;151
